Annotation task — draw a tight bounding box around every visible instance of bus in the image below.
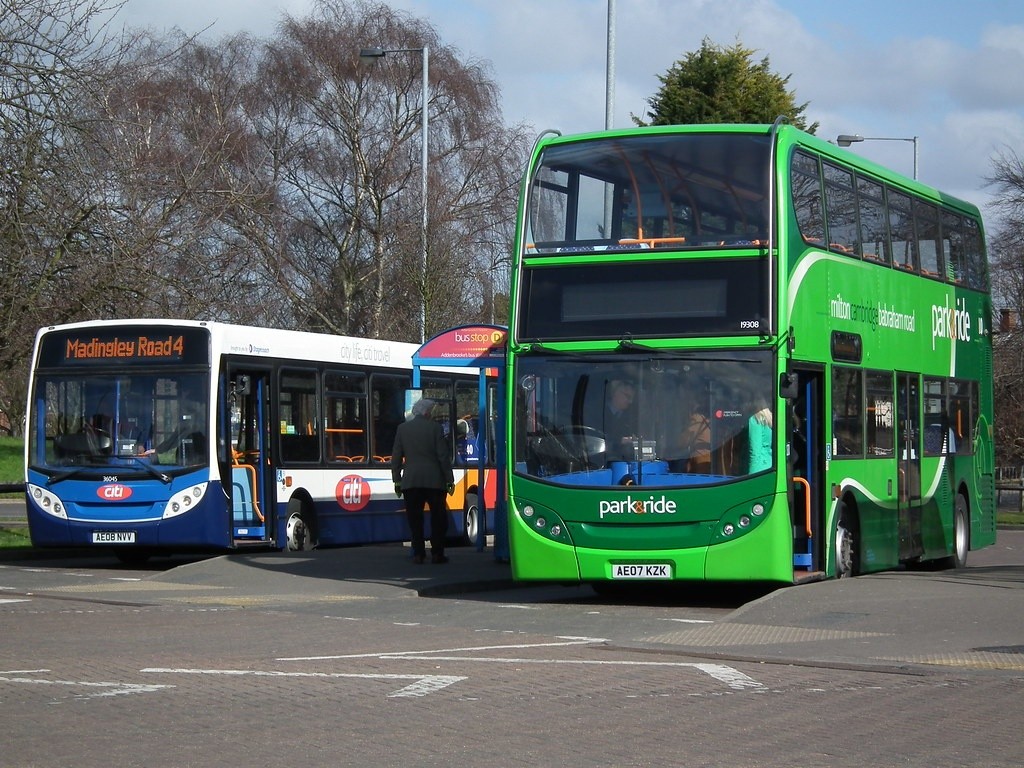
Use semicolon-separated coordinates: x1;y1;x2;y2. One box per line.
23;317;504;563
501;115;998;590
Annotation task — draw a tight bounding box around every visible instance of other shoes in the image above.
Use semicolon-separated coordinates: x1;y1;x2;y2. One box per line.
411;553;449;567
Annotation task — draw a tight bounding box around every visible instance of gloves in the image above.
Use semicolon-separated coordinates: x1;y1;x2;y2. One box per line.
446;481;455;496
395;482;402;498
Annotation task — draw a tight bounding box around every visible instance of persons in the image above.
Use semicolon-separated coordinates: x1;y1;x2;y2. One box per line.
85;370;208;464
390;397;456;565
586;375;637;462
654;364;773;475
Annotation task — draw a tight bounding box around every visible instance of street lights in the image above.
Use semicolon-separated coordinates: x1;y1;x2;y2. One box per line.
356;46;430;346
833;135;918;181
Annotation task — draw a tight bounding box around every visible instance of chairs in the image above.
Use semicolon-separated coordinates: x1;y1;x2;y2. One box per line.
333;454;406;464
802;232;962;286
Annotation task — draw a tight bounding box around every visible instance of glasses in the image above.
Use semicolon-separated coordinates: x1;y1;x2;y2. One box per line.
619;388;638;401
